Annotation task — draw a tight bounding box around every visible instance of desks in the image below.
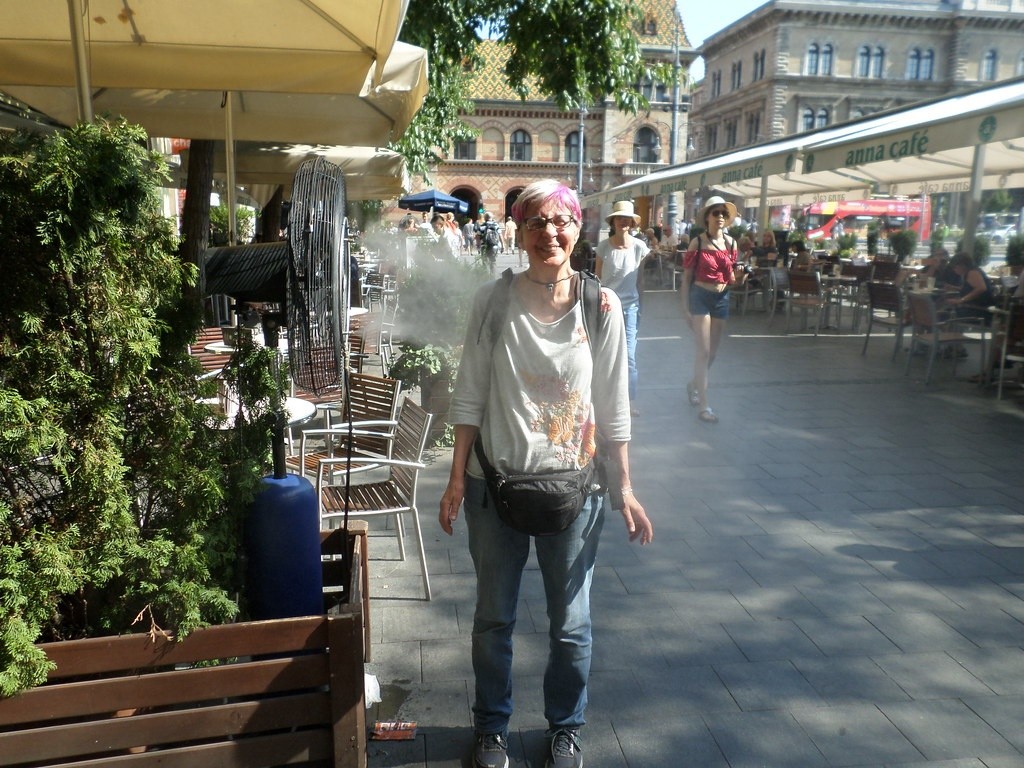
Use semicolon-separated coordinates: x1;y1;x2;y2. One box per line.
822;274;857;331
203;335;301;355
193;397;317;435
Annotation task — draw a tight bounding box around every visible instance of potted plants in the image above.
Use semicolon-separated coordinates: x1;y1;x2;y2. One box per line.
383;229;496;450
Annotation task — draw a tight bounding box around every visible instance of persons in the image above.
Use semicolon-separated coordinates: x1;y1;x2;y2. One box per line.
437;180;653;768
645;207;1024;368
398;211;523;275
595;202;650;415
680;195;739;423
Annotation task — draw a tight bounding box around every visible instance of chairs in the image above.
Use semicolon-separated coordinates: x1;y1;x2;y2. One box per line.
185;262;435;604
643;249;1024;401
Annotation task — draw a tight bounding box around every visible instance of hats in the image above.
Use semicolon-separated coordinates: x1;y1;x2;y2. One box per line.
605;201;641;227
697;195;738;228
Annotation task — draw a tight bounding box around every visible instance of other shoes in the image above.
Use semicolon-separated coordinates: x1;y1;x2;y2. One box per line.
630;408;639;416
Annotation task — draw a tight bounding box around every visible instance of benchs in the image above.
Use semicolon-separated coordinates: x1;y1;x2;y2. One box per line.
307;305;369;318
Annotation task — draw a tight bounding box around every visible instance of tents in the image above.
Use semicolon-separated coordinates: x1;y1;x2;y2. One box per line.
0;0;430;202
580;81;1024;211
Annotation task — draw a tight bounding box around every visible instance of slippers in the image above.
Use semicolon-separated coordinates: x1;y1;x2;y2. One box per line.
697;407;718;423
686;381;700;407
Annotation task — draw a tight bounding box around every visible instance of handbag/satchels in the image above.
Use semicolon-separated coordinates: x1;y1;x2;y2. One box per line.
485;471;589;536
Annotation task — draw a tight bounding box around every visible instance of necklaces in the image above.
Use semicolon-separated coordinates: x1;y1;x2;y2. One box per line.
525;270;575;293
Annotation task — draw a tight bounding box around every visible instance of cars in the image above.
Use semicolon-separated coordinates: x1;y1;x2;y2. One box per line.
962;211;1020;245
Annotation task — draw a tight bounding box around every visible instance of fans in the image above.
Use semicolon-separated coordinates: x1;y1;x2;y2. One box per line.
203;155;350;621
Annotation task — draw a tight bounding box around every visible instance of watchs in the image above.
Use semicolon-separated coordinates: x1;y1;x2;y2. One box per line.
960;298;965;302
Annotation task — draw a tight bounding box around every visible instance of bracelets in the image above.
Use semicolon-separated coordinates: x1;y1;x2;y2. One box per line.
620;485;633;497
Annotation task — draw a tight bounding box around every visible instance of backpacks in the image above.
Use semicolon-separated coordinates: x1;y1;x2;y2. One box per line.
481;222;500;246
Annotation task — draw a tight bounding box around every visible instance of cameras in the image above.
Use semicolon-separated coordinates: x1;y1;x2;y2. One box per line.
731;262;753;272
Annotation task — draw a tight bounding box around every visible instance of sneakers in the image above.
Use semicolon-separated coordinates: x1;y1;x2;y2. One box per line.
543;724;584;768
472;732;510;768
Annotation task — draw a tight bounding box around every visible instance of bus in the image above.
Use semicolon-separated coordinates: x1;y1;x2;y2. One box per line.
798;197;932;244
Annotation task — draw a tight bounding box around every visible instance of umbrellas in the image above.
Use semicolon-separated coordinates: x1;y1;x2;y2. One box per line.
398;189;469;217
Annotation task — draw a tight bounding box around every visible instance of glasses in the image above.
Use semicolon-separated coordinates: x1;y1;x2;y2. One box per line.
524;215;574;231
711;209;729;217
